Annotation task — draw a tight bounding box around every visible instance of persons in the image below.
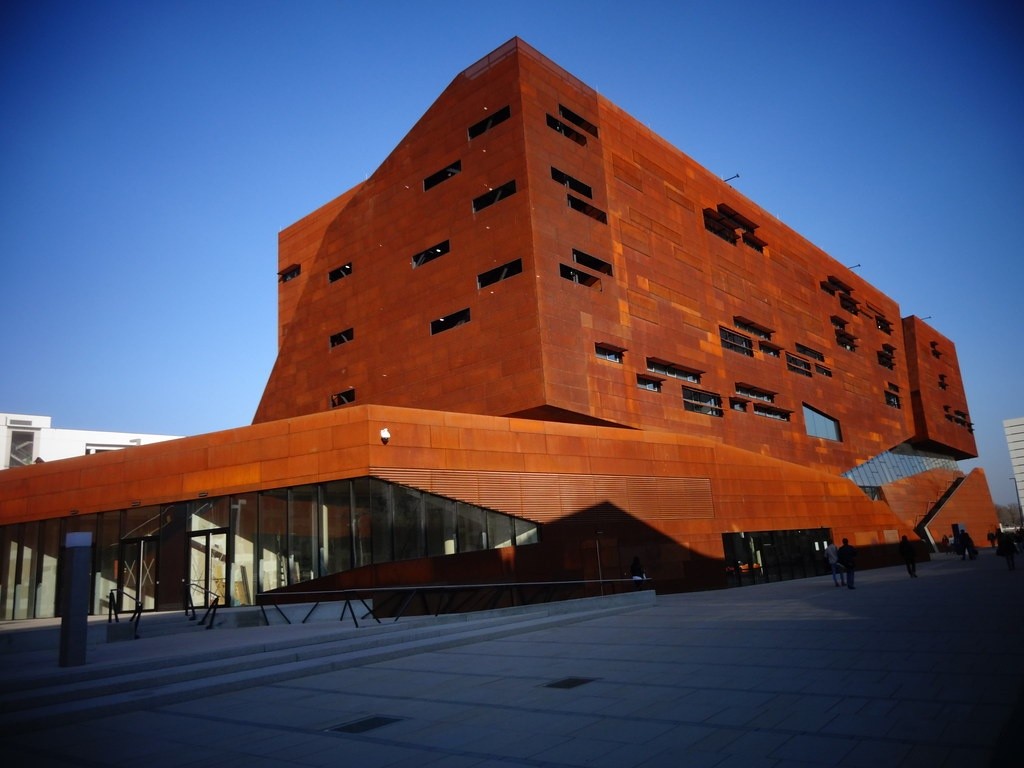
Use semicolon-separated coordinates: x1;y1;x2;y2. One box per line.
959;530;977;560
823;538;857;589
987;527;1024;572
898;535;918;578
942;534;956;555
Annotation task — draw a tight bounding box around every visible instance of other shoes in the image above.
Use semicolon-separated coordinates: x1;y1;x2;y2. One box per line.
1009;568;1014;571
910;574;918;577
836;583;841;587
842;582;847;586
836;560;845;570
848;585;856;589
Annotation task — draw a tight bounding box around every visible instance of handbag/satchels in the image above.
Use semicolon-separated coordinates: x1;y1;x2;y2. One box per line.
996;548;1005;557
971;549;978;555
